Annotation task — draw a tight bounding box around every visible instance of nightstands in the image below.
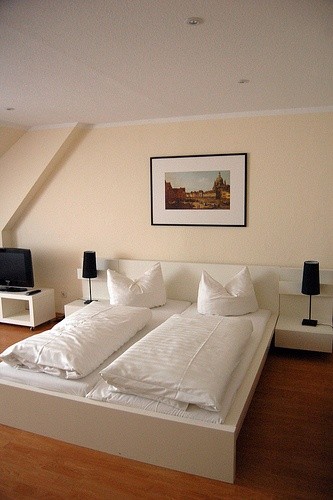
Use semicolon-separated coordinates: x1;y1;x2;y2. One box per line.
274;268;333;353
63;259;107;317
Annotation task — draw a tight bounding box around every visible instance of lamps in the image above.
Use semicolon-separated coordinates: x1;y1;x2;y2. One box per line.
83;251;98;304
301;260;320;327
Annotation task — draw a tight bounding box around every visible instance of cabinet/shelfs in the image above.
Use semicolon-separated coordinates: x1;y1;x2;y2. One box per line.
0;285;56;331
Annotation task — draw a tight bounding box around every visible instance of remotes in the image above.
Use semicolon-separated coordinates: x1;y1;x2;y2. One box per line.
26;290;41;295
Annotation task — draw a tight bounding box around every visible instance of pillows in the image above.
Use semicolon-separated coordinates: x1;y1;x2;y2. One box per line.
197;266;259;316
107;262;167;308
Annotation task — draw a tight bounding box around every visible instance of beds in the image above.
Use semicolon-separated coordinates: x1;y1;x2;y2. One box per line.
0;259;280;484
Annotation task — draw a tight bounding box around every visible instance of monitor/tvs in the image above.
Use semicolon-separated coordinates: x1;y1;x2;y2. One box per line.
0;247;35;292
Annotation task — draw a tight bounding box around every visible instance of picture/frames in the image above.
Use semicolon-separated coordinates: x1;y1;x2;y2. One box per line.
150;153;247;227
